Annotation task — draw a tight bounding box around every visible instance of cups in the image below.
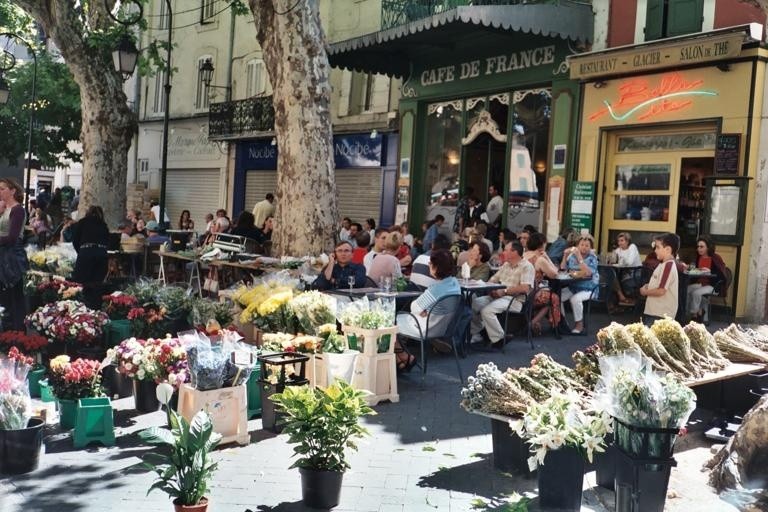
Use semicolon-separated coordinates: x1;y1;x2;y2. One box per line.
379;274;397;291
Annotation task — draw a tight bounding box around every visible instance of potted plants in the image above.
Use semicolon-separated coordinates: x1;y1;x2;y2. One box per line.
137;407;223;511
266;377;379;507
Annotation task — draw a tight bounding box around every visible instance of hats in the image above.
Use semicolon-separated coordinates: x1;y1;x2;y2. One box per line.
144;220;159;231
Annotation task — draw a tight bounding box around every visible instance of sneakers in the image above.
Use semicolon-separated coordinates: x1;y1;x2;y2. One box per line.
429;338;452;357
529;320;540;337
619;298;635;305
608;305;624;314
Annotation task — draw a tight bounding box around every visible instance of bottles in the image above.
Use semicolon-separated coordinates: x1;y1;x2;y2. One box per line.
625;173;708;208
567;252;576;269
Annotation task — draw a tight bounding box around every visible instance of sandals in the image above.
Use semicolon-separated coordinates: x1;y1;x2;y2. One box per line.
397;354;417;373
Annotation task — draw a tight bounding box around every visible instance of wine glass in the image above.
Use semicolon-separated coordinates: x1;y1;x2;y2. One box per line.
347;275;355;293
462;262;471;285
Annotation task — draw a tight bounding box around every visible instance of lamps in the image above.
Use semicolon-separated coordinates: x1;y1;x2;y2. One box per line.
199;58;229;97
369;129;400;140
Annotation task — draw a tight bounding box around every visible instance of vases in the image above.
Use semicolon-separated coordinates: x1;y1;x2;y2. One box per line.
0;419;46;476
534;447;585;511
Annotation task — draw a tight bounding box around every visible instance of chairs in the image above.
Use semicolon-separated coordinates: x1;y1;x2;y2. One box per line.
365;263;644;384
701;267;733;327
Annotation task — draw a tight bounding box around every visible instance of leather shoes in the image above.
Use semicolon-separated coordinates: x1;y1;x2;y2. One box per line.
572;327;583;334
698;308;705;323
691;313;698;323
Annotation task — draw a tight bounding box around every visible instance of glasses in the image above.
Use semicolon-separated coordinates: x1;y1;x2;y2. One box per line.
698;245;706;249
334;249;351;253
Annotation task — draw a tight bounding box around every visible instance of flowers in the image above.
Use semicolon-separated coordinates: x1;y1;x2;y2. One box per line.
457;313;728;454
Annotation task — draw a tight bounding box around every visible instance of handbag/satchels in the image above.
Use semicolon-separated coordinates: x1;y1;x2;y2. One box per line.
203;278;219;293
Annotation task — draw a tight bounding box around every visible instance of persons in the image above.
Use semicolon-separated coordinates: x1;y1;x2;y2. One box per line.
0;177;27;337
120;200;170;242
611;232;726;328
312;215;635;373
178;192;276;246
70;205;111;309
461;184;504;240
28;184;80;244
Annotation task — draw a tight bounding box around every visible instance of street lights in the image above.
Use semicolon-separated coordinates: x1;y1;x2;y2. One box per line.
0;31;40;224
103;1;175;232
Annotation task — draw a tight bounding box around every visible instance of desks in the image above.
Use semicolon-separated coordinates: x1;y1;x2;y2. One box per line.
327;286;424;312
678;267;712;328
166;229;197;248
